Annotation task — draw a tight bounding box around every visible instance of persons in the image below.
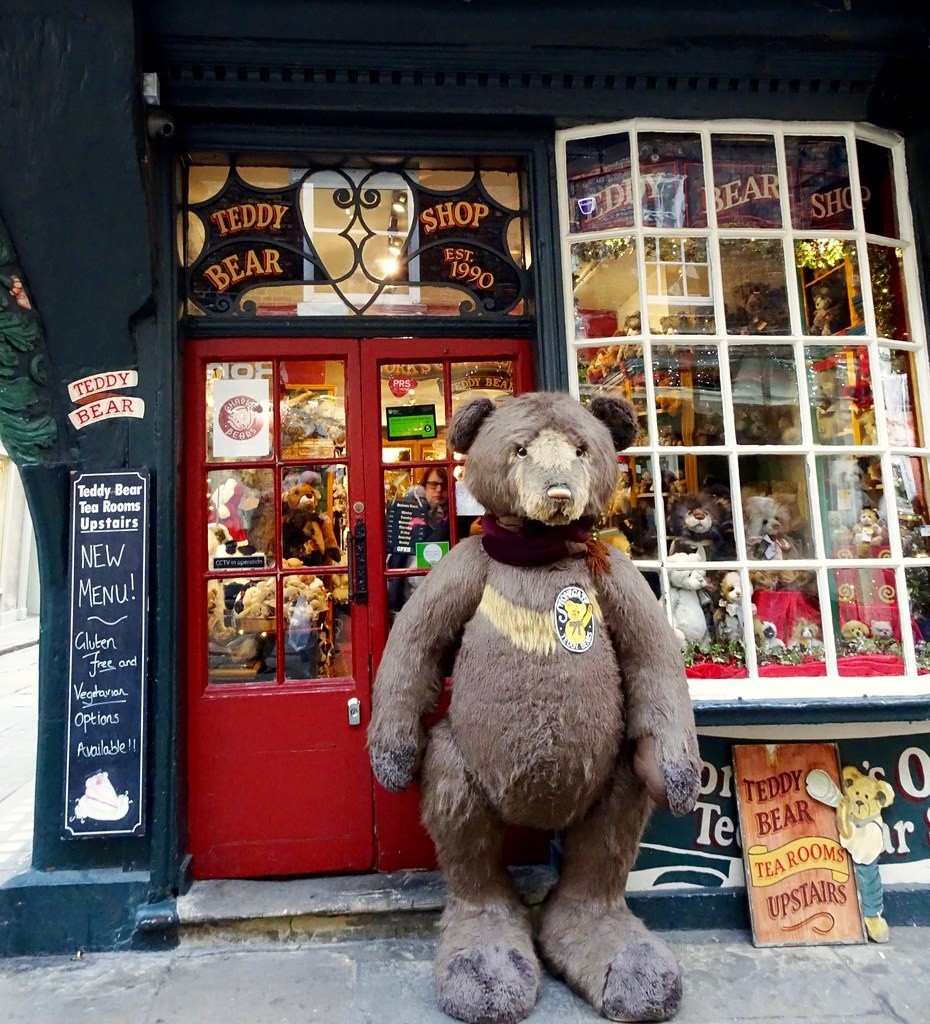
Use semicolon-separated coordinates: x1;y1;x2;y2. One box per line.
385;467;482;627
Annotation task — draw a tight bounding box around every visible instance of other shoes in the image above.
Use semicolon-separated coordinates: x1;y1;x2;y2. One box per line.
328;643;340;653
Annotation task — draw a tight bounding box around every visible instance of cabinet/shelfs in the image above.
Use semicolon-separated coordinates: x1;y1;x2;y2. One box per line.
847;349;875;459
798;255;864;336
610;371;699;508
283;384;339;649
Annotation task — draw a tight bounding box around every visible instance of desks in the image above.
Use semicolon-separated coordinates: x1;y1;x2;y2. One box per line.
208;660;262;682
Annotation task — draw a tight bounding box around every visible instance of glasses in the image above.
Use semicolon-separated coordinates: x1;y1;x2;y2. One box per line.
425;480;448;490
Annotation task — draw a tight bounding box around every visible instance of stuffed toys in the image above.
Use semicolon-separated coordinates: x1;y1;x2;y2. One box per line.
206;370;347;681
585;278;899;657
366;392;699;1024
